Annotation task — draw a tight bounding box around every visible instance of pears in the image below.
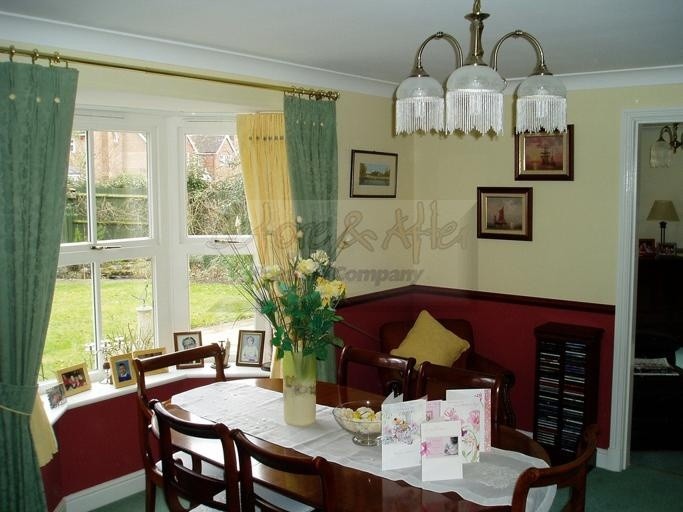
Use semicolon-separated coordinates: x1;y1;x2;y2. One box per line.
353;412;380;432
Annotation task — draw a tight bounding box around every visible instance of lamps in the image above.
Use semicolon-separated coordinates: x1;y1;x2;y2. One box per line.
646;200;680;256
392;0;567;137
650;122;683;168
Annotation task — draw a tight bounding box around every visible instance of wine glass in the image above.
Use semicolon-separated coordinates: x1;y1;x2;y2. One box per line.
331;399;384;448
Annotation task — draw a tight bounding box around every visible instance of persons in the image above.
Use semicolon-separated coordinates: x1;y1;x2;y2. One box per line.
69;375;74;389
72;373;82;387
77;373;83;381
241;336;258;362
117;363;131;380
180;336;199;363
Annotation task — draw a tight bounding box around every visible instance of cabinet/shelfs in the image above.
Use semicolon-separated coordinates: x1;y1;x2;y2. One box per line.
635;256;683;358
532;323;605;469
630;367;683;451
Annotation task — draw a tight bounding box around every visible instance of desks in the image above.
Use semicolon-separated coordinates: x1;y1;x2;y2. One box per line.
152;375;559;512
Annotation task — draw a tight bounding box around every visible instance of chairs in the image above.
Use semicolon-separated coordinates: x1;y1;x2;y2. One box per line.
636;319;683;366
337;344;416;401
147;398;240;512
513;426;601;512
131;344;226;511
413;361;509;424
227;430;335;512
381;318;518;429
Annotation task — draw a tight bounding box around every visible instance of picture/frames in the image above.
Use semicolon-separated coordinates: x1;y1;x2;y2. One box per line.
638;238;655;253
350;150;399;198
514;124;574;182
36;330;265;409
476;186;533;241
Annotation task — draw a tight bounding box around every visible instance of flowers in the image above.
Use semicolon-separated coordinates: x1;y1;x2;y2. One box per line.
200;205;347;361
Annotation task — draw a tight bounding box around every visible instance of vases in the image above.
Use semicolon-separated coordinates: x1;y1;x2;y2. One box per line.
281;350;317;427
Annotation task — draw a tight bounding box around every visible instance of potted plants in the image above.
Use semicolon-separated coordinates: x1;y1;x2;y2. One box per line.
130;279;152;350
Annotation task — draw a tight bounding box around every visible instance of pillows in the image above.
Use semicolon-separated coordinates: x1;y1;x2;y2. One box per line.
389;310;472;373
